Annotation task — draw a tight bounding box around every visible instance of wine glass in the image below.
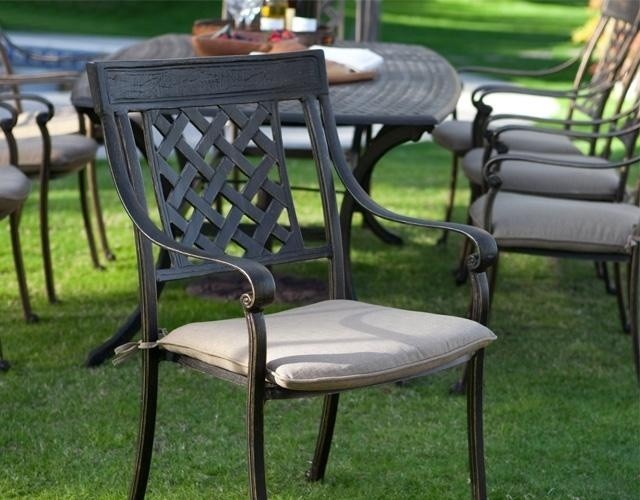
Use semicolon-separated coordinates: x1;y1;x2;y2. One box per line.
225;0;264;31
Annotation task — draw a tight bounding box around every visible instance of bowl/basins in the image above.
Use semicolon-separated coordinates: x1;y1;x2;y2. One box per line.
192;29;297;59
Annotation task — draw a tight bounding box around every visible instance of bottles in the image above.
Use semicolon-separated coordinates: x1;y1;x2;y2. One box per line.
259;5;286;32
294;0;318;31
